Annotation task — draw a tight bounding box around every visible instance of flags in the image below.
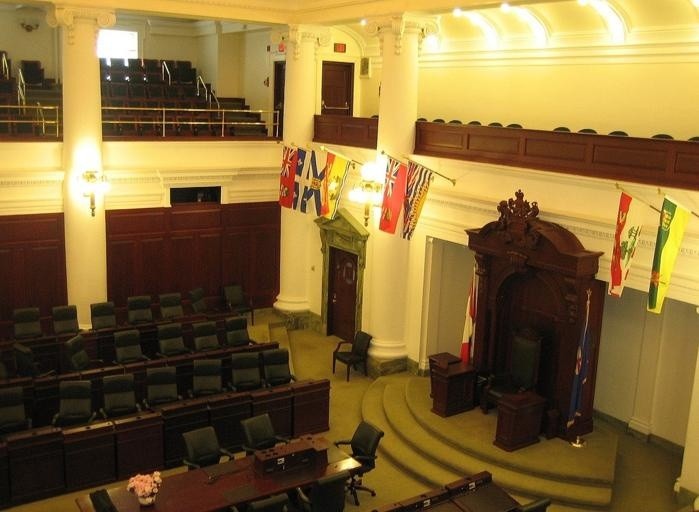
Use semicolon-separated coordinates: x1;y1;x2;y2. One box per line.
608;191;651;298
459;262;482;370
561;287;593;440
278;145;298;209
299;150;323;216
379;157;408;235
293;148;312;212
646;193;690;314
320;151;350;220
399;161;434;240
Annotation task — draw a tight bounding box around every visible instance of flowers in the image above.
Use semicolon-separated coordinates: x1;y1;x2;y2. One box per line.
126;470;163;499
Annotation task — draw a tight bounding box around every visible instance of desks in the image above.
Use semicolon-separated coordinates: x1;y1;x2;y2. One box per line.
0;377;331;512
0;306;239;386
371;469;521;512
0;339;280;435
74;435;363;512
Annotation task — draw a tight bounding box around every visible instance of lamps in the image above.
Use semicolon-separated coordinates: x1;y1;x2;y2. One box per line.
71;136;115;218
346;147;390;228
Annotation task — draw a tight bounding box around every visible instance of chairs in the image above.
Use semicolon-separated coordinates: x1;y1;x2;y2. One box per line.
190;320;222;351
447;119;463;124
181;425;235;471
223;315;258;350
126;100;157;134
433;119;446;123
515;495;553;512
160;59;179;82
110;58;128;82
157;292;186;319
99;372;142;420
100;57;111;80
178;100;193;132
127;58;145;81
239;412;290;456
51;380;96;426
160;100;177;133
371;114;377;118
194;100;212;132
143;365;183;410
688;136;698;141
468;120;482;126
12;306;47;340
143;58;161;83
20;59;46;89
332;330;373;382
228;351;267;391
51;304;85;335
487;122;502;126
221;283;254;327
505;123;522;128
332;419;386;507
0;386;33;437
126;295;156;324
176;59;197;83
145;82;166;99
187;358;227;399
261;347;299;388
156;321;195;360
101;80;110;98
552;126;570;132
129;83;146;98
177;85;195;99
112;329;151;365
607;130;628;136
90;301;122;330
145;99;162;136
64;332;106;372
186;287;220;313
577;128;597;134
417;117;426;121
165;84;179;99
479;324;546;415
651;133;673;139
11;340;59;379
107;99;138;134
110;81;128;98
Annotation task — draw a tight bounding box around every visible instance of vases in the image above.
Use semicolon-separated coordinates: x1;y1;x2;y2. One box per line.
138;494;157;506
